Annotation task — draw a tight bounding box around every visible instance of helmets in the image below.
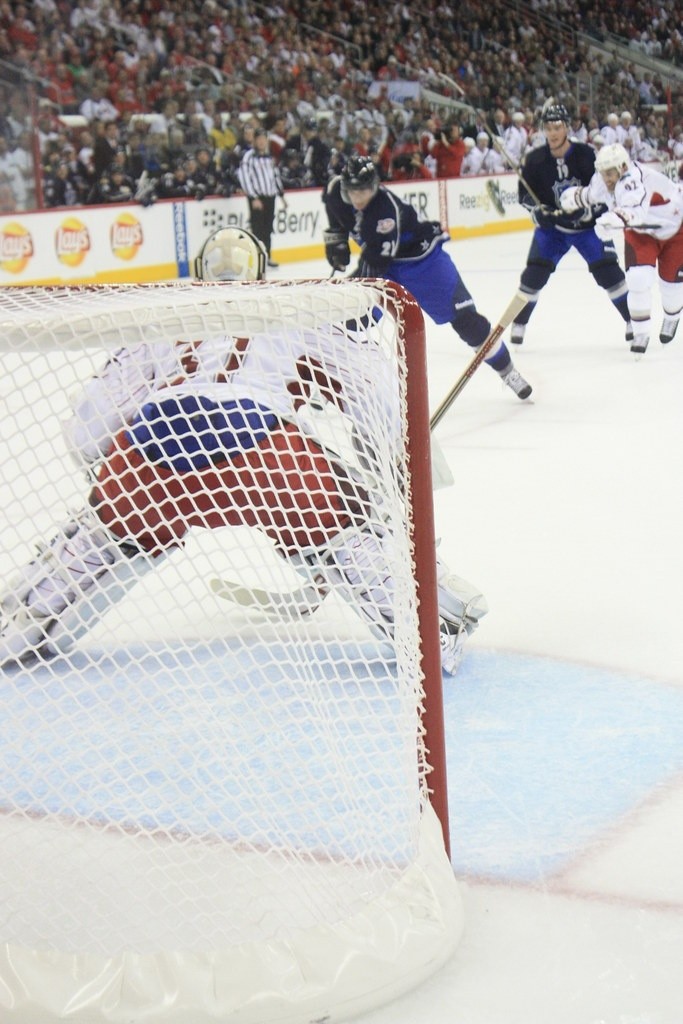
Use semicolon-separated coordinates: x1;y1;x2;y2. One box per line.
194;225;267;281
593;143;633;178
340;154;379;204
253;127;268;139
542;104;569;127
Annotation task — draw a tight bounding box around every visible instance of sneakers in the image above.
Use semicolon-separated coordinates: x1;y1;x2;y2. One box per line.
624;321;634;346
509;323;525;354
630;334;651;361
658;318;680;348
502;370;534;404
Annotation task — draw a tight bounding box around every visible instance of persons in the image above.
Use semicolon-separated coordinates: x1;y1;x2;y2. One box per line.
320;156;536;403
459;132;507;176
0;227;491;677
93;121;130;177
559;143;683;362
518;17;538;45
77;87;121;122
638;72;660;104
508;104;635;355
0;0;683;214
237;128;288;267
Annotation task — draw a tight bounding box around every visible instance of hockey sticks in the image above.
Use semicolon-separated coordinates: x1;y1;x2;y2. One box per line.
437;71;547;214
210;293;529;621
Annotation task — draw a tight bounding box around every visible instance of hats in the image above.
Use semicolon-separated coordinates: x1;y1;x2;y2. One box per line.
607;112;619;124
511;112;525;122
621;111;632;121
463;136;475;148
476;131;490;141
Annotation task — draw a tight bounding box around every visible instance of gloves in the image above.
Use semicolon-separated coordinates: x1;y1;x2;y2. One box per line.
559;185;584;215
529;203;556;231
594;208;631;242
323;229;350;272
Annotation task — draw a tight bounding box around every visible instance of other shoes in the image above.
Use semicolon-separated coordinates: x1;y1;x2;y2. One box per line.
268;259;279;267
